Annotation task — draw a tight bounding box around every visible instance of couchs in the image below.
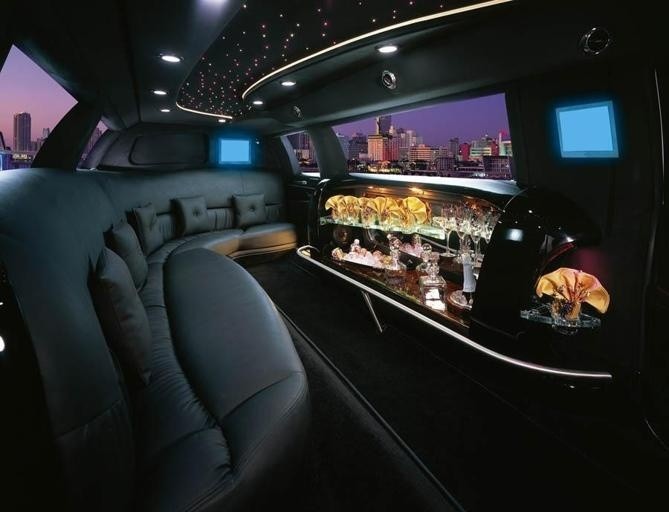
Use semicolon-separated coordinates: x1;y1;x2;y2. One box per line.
1;167;315;512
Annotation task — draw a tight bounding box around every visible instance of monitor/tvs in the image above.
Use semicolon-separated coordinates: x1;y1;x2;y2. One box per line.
556;101;622;161
218;138;252;165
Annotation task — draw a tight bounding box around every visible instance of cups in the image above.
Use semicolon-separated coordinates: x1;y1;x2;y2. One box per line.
553;297;583;327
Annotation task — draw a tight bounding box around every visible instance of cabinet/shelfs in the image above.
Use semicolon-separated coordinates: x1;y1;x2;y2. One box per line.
297;172;615;395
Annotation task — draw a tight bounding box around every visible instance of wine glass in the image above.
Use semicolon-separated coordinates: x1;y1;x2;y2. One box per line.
440;204;496;270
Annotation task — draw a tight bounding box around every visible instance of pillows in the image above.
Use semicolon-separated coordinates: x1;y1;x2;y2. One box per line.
93;201;164;387
231;193;268;229
172;195;212;237
